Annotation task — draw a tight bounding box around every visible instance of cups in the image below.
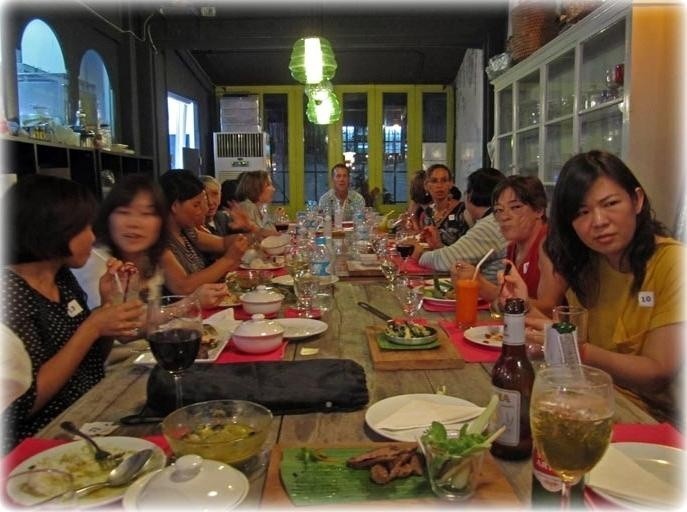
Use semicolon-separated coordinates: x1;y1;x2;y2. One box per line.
238;270;257;293
334;239;343;256
274;215;289;232
552;306;589;364
456;269;478;326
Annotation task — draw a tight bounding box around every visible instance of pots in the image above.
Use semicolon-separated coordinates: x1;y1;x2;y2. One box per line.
358;303;439;343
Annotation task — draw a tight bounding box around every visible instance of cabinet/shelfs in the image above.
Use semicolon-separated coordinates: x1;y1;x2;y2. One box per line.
0;133;158;202
490;0;687;243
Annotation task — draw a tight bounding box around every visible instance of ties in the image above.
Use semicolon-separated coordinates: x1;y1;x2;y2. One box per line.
205;222;218;235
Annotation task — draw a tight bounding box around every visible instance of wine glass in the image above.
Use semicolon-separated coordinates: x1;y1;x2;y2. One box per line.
146;297;203;410
372;230;426;323
293;270;321;319
529;361;615;511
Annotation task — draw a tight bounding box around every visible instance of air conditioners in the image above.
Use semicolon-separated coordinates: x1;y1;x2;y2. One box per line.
213;131;271;185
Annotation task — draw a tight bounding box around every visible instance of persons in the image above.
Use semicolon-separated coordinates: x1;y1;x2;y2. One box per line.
401;163;570;325
498;150;687;410
318;164;365;221
0;169;286;457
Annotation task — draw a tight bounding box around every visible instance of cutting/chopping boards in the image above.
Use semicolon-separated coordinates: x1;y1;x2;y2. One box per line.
345;260;384;276
365;325;466;370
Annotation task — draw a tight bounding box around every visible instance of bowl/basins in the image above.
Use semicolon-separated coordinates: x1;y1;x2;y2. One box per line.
261;235;291;256
230;328;282;353
122;399;273;512
239;292;285;315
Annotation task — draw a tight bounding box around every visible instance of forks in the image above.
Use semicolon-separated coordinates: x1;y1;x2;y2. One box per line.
59;420;118;471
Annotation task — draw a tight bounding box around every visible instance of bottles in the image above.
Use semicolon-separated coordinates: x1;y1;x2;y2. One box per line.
492;298;585;511
295;200;344;313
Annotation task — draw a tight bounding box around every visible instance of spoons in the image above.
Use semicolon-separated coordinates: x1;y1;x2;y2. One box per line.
18;448;155;507
489;263;513;322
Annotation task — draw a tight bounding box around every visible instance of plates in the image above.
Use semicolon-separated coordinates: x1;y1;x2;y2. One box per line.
239;255;285;270
465;325;505;347
133;318;243;368
414;283;460;302
363;393;489;442
272;274;340;285
586;441;687;511
273;319;328;340
5;436;169;509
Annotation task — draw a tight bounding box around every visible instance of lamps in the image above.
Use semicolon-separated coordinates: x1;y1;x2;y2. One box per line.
305;81;334;101
288;36;338;84
305;93;341;125
